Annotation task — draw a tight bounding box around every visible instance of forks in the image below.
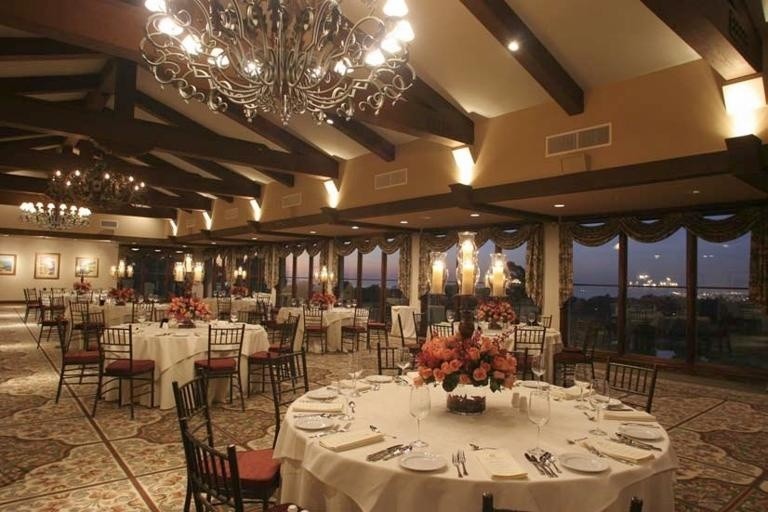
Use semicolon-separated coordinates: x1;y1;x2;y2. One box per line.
449;450;469;478
309;423;352;437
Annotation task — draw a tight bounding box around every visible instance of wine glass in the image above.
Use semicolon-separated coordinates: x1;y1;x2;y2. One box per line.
291;296;360;311
347;353;365;397
447;309;536;330
138;293;160;306
408;385;432;447
571;362;593;401
211;287;256;303
530;353;548;392
133;305;240;331
397;346;411;385
528;390;551;454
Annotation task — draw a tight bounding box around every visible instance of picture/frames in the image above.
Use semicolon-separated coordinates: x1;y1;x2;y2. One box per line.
75;256;98;278
0;254;16;275
34;252;60;279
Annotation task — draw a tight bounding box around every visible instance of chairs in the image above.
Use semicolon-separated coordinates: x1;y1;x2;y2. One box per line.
705;308;732;357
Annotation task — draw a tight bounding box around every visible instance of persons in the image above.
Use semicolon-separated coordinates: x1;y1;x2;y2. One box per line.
508;261;524;282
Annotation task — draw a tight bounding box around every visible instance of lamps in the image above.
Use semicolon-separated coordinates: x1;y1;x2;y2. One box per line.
483;253;511;297
427;252;449;296
455;230;481;297
137;0;416;128
725;134;768;175
447;183;473;208
17;194;95;237
42;149;147;213
108;206;339;283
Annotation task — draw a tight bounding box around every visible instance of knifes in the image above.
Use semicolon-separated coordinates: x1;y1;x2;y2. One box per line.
367;444;413;461
525;449;565;478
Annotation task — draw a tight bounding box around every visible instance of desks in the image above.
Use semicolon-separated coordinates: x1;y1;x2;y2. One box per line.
734;305;768;333
664;315;711;339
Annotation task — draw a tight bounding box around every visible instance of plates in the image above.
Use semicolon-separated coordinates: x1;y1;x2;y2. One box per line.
308;389;338;401
594;393;622;408
617;424;662;442
175;333;189;337
296;416;333;432
247;326;261;330
402;452;447;473
560;451;610;472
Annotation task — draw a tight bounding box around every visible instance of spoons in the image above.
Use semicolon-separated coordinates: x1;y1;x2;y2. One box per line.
348;401;356;416
